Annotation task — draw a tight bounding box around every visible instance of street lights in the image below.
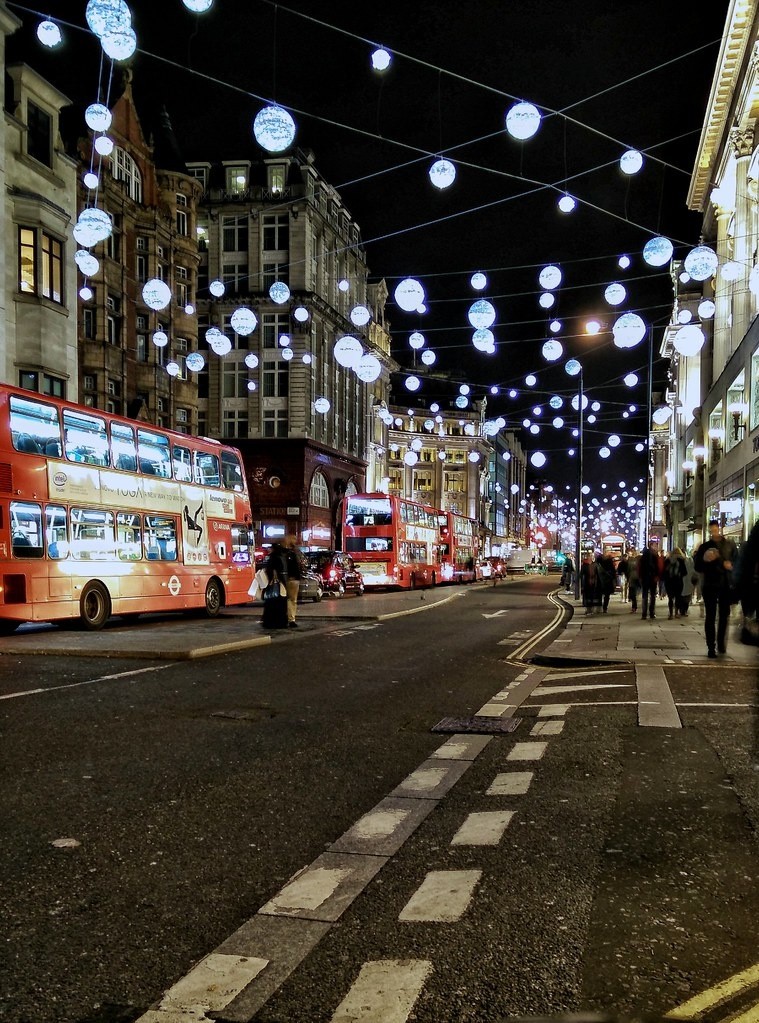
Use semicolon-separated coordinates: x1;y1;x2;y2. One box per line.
583;318;655;549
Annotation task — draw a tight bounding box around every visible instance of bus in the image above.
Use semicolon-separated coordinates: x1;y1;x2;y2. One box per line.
1;381;257;636
335;492;481;593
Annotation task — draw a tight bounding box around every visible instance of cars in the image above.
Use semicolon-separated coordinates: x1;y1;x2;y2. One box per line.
543;557;562;573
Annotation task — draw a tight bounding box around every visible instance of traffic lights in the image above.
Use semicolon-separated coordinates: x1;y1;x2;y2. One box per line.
557;555;563;561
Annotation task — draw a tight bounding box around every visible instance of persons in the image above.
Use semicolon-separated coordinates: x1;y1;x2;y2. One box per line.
693;520;740;658
619;542;697;619
739;518;759;618
183;501;203;544
582;547;617;614
266;534;304;626
562;553;575;591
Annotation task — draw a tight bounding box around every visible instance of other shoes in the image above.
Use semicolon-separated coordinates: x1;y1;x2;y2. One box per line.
630;608;637;614
289;621;297;628
675;613;680;619
668;615;673;620
603;608;608;613
564;590;573;595
717;648;726;654
679;609;686;616
641;616;646;620
650;615;656;618
708;653;719;659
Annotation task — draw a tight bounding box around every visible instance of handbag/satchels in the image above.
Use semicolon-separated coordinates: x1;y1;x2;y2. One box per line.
261;578;287;601
246;569;270;601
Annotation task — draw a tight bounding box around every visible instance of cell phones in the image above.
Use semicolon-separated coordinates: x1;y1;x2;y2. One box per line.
709;548;716;553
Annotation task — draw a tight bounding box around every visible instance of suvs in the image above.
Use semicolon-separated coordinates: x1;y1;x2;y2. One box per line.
242;545;324;603
303;549;365;599
481;556;508;580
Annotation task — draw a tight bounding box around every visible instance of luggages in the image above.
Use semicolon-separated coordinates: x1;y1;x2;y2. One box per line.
261;586;288;630
